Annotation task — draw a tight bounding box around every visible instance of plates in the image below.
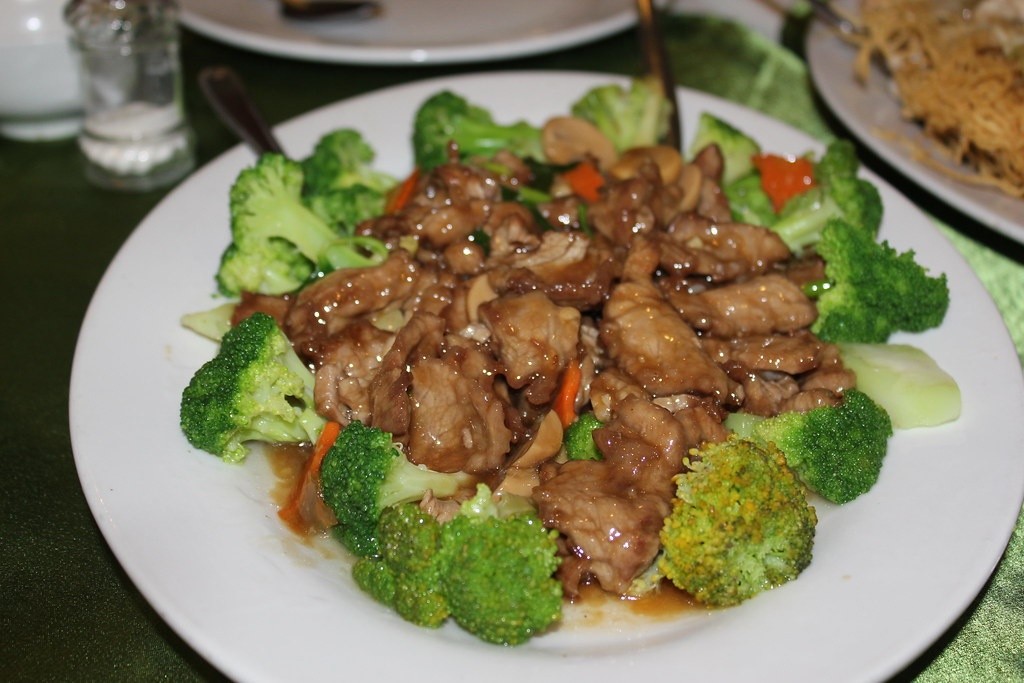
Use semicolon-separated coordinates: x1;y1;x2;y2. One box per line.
176;0;640;67
68;69;1023;683
804;0;1024;245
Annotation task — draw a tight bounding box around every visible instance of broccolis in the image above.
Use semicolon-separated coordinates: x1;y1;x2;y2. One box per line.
178;83;948;646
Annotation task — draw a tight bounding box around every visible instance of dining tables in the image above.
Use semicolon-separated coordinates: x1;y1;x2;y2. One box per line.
1;14;1023;683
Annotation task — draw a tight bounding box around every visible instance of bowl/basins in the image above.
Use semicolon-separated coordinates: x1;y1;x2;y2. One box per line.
0;0;122;141
